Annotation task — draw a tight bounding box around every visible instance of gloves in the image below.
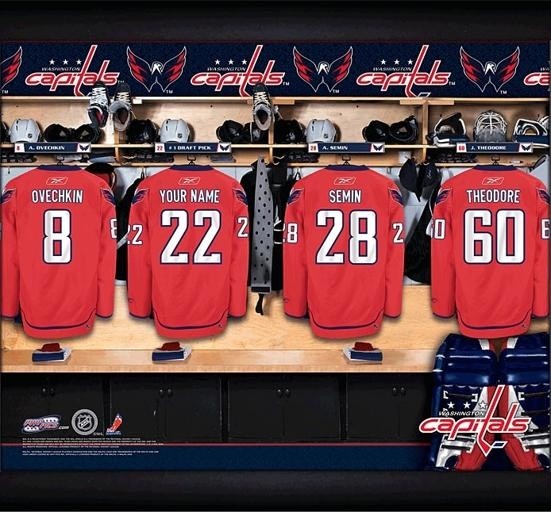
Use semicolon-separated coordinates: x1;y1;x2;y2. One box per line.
216;120;246;144
390;116;419;144
243;121;269;144
362;120;390;144
44;124;72;142
73;125;105;144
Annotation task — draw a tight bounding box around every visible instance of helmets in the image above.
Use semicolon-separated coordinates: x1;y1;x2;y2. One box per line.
473;111;507;143
126;118;158;144
274;119;306;144
158;119;192;143
8;119;43;143
426;112;470;148
305;119;336;143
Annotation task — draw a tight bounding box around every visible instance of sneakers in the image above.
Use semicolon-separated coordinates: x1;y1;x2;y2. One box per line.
109;82;134;131
252;82;273;131
88;81;109;127
